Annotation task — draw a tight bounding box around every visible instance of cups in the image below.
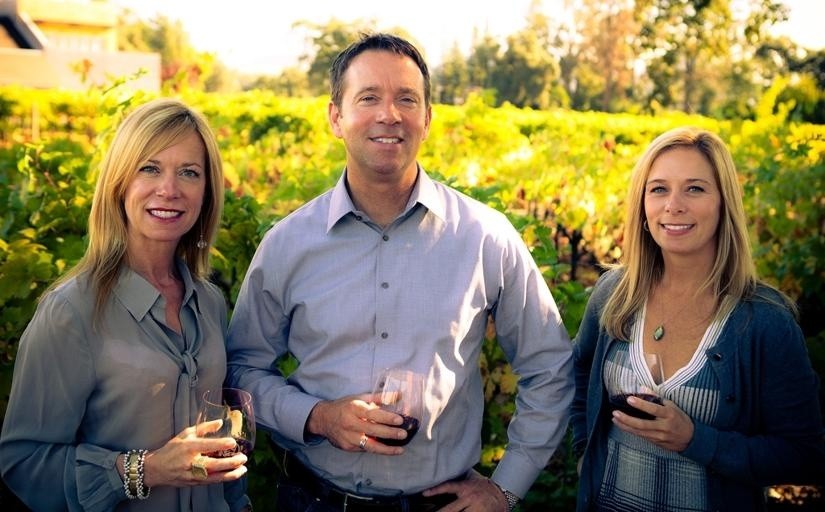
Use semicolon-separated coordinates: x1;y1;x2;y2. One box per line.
609;350;665;421
197;387;257;472
367;368;427;448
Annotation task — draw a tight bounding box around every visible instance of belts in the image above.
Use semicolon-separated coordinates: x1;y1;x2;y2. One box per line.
266;435;468;511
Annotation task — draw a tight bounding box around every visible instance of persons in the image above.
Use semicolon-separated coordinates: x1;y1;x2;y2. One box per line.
225;34;576;512
2;100;248;512
571;128;825;512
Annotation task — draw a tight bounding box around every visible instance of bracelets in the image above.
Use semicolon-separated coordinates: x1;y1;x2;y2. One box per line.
123;448;151;500
501;488;518;511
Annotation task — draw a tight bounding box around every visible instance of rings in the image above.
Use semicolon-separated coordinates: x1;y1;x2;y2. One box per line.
191;456;208;481
359;436;368;449
654;277;708;341
